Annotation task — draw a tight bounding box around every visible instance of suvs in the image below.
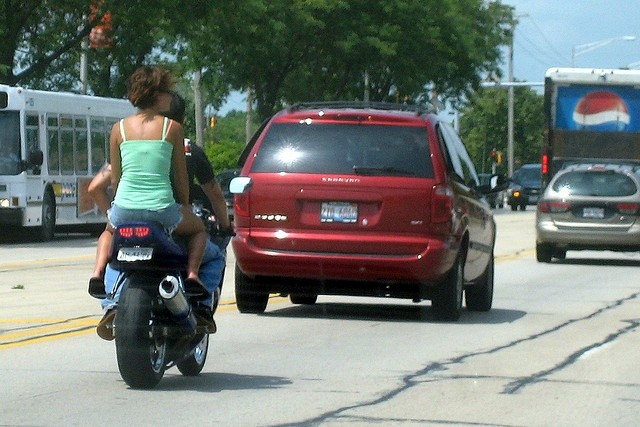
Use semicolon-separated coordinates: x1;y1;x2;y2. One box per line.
511;163;542;211
229;98;507;321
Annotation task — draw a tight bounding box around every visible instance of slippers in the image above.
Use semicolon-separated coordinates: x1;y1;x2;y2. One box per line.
183;279;208;299
89;278;107;300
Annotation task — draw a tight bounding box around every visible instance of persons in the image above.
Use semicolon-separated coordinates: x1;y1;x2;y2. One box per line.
88;65;213;303
88;91;237;340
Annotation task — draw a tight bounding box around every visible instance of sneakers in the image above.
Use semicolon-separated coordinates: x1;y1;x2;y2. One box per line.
197;304;216;333
97;302;117;323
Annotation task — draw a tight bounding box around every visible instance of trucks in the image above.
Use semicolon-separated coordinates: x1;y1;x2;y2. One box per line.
540;66;640;198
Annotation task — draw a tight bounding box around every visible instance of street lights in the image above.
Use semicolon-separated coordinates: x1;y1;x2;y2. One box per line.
570;35;636;66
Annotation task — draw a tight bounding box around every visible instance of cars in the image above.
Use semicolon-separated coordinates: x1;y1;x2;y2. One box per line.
477;173;504;208
535;160;640;263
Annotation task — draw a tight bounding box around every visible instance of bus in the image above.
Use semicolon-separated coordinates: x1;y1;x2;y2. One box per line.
0;83;138;242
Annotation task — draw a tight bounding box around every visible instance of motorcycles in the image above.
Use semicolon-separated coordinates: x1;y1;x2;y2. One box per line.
102;182;236;387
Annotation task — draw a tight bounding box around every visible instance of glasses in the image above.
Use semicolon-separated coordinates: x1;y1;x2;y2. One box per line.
156;88;177;95
